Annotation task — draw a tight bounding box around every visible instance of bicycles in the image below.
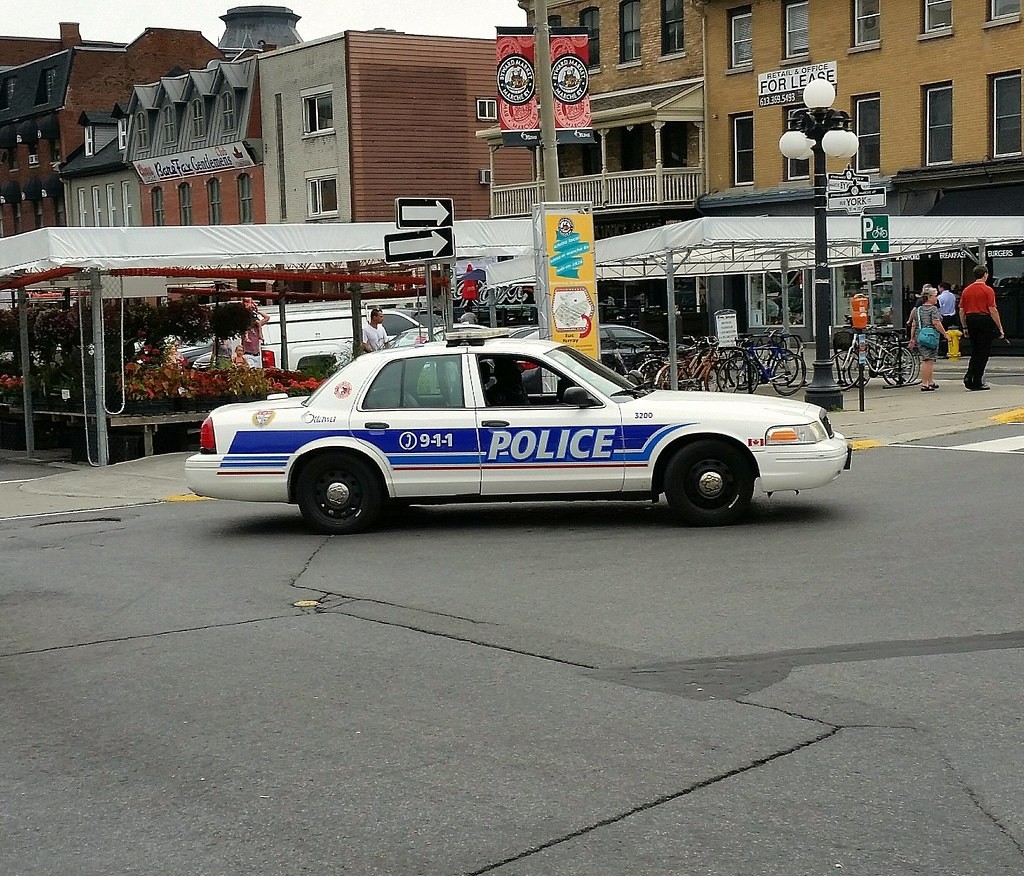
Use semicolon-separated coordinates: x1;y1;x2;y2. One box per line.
829;312;927;392
605;327;808;398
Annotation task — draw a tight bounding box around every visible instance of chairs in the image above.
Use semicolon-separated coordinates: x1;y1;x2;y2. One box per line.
477;357;532;408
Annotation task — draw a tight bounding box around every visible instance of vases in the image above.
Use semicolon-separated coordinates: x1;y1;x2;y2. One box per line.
125;397;271;414
3;396;22;405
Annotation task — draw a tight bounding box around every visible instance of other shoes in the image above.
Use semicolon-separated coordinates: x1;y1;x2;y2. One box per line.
964;377;972;389
972;384;991;391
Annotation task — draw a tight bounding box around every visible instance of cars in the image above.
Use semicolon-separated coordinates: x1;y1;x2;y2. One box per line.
511;325;690;398
382;324;492;354
182;325;855;533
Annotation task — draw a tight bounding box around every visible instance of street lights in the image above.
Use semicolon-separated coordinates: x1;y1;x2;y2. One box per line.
779;76;863;413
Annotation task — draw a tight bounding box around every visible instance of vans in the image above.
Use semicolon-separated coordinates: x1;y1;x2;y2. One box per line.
139;302;441;375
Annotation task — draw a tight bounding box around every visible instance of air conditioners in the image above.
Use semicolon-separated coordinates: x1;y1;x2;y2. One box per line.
480;169;491;185
28;155;39;164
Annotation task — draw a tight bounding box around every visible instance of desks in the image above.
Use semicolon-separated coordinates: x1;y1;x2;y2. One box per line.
109;414;207;458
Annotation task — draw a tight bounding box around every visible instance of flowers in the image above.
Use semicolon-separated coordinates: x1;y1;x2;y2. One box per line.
114;360;326;397
0;373;24;394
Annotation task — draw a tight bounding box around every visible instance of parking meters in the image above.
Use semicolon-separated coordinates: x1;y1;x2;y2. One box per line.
852;291;868;412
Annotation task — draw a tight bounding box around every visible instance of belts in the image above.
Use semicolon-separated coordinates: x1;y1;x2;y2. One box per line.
966;314;987;316
245;352;260;356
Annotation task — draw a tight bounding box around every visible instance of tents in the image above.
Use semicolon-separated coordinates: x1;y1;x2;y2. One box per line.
486;213;1024;390
0;213;536;466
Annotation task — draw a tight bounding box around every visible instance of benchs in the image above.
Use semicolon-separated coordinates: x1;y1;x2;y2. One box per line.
363;386;418;412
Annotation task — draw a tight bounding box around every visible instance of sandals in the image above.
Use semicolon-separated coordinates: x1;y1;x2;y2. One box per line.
921;385;935;391
929;383;939;388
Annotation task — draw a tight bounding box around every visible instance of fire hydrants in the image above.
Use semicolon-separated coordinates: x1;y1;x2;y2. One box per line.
945;329;963;362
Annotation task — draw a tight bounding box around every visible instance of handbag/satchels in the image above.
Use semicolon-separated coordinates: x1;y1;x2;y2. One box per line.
989;318;1001;339
917;327;938;350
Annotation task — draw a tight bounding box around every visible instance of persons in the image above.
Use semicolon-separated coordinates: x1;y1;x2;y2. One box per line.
458;305;478;324
234;298;270;368
166;339;184;368
208;336;232;371
363;308;390;353
906;282;961;392
957;265;1004;391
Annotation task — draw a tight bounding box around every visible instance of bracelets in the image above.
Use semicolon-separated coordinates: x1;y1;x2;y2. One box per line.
963;327;968;330
